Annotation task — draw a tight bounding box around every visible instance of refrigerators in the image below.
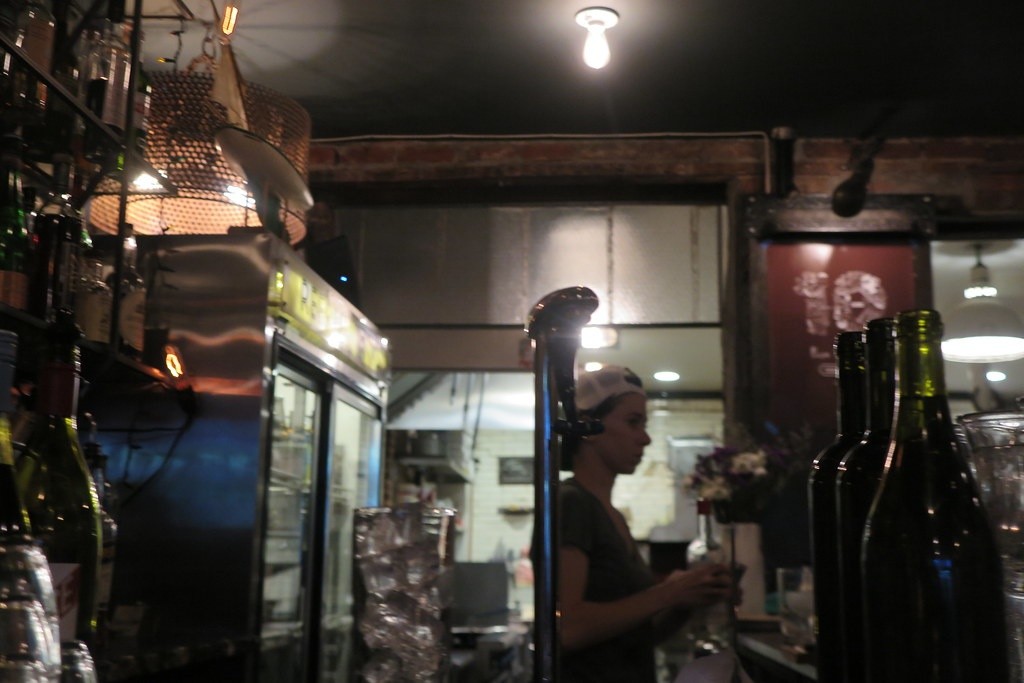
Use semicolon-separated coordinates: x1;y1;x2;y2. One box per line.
146;231;389;681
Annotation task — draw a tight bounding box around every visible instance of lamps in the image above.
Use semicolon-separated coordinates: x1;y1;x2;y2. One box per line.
940;302;1024;364
964;245;997;298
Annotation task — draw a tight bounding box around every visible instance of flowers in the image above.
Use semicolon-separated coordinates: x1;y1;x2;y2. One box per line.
691;422;810;502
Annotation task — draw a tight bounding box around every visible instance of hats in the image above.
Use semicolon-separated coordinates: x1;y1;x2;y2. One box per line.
557;366;647;419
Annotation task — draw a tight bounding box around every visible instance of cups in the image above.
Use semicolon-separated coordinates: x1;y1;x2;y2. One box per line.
951;413;1023;599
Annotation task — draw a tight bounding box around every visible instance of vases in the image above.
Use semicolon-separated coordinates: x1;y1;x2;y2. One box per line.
714;497;774;523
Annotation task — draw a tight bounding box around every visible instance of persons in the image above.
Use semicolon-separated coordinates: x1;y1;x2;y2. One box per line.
528;365;732;683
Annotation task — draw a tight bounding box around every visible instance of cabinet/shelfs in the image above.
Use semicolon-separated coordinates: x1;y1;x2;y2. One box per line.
0;0;143;683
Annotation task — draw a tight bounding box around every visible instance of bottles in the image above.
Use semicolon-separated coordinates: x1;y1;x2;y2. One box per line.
835;317;900;678
809;331;872;683
1;2;164;682
862;309;1010;683
687;498;735;653
